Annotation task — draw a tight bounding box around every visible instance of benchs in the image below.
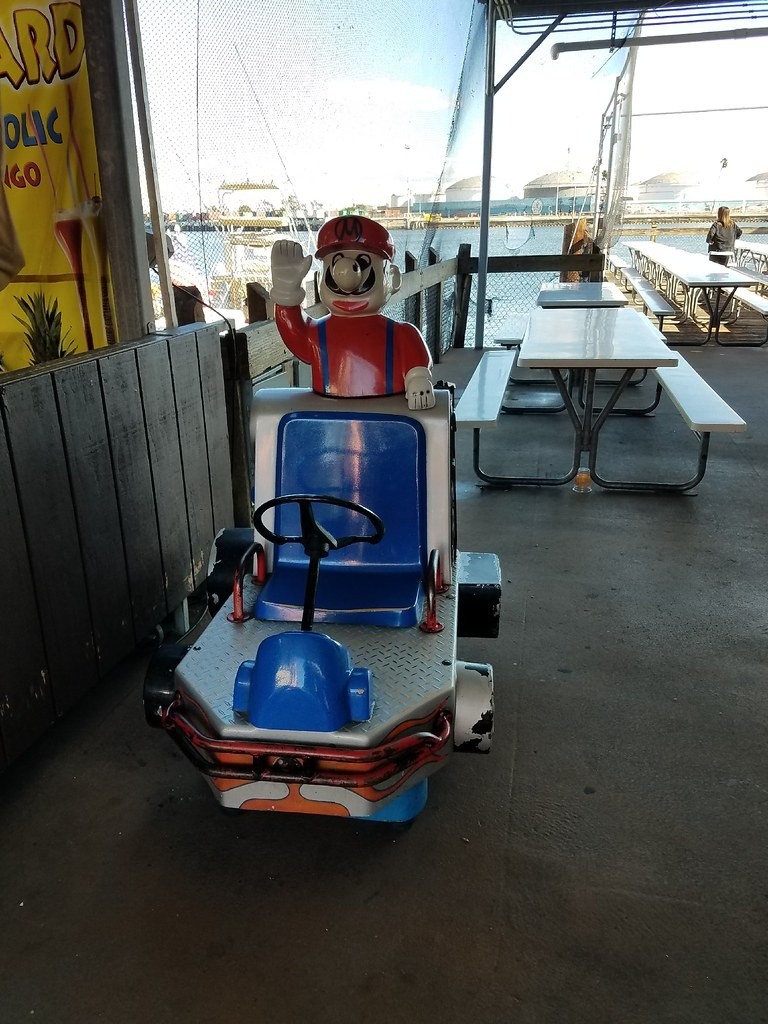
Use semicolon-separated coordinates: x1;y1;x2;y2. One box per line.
493;312;530;384
636;310;668;385
717;262;768;347
606;254;681;347
454;349;516;485
650;350;747;492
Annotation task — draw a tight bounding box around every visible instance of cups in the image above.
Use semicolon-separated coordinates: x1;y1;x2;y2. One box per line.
572;467;593;494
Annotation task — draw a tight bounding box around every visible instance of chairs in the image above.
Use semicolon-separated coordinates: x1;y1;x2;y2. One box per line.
252;411;427;628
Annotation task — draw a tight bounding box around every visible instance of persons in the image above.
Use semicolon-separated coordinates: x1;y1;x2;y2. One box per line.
269;214;435;411
706;205;743;265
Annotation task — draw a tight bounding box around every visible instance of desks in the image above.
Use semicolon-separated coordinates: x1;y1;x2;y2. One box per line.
528;281;639;387
621;242;759;347
732;239;768;274
516;308;679;491
